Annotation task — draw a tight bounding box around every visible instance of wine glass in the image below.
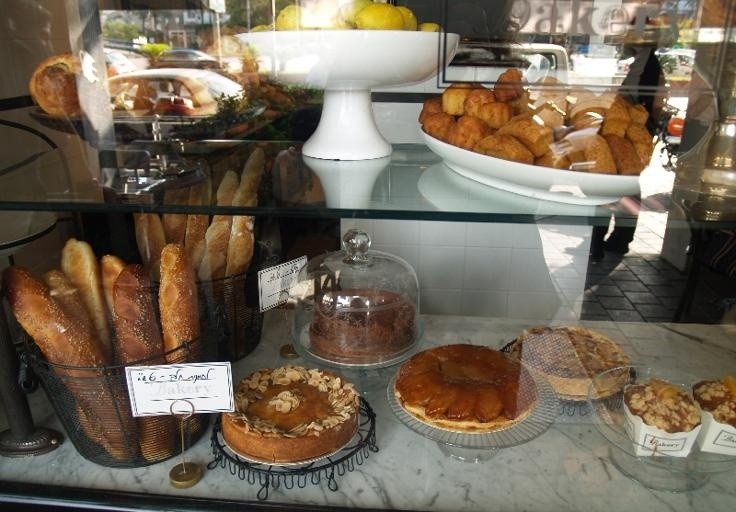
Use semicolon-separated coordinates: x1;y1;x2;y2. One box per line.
586;364;732;492
27;100;267;194
233;29;462;162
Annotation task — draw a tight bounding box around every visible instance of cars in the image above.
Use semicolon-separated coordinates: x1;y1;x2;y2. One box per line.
105;41;261;139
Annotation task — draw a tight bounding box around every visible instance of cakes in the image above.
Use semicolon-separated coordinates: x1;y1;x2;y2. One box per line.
505;326;630;398
623;379;701;434
693;375;735;431
219;367;360;463
394;344;538;432
309;288;414;365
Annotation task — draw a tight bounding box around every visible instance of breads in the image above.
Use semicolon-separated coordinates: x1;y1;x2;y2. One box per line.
1;244;204;462
418;67;652;178
127;150;265;361
27;50;217;118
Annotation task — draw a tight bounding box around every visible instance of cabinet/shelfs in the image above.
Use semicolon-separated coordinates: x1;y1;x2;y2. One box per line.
0;94;736;512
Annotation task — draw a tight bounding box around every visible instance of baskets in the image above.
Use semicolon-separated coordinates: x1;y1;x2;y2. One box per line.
19;284;229;468
148;240;272;365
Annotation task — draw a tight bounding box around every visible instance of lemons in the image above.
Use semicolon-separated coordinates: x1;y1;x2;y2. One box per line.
248;1;443;32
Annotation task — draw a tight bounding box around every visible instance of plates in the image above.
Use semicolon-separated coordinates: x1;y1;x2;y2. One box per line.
393;384;530;433
420;124;656;206
415;159;602;213
300;316;427;368
221;420;360;466
